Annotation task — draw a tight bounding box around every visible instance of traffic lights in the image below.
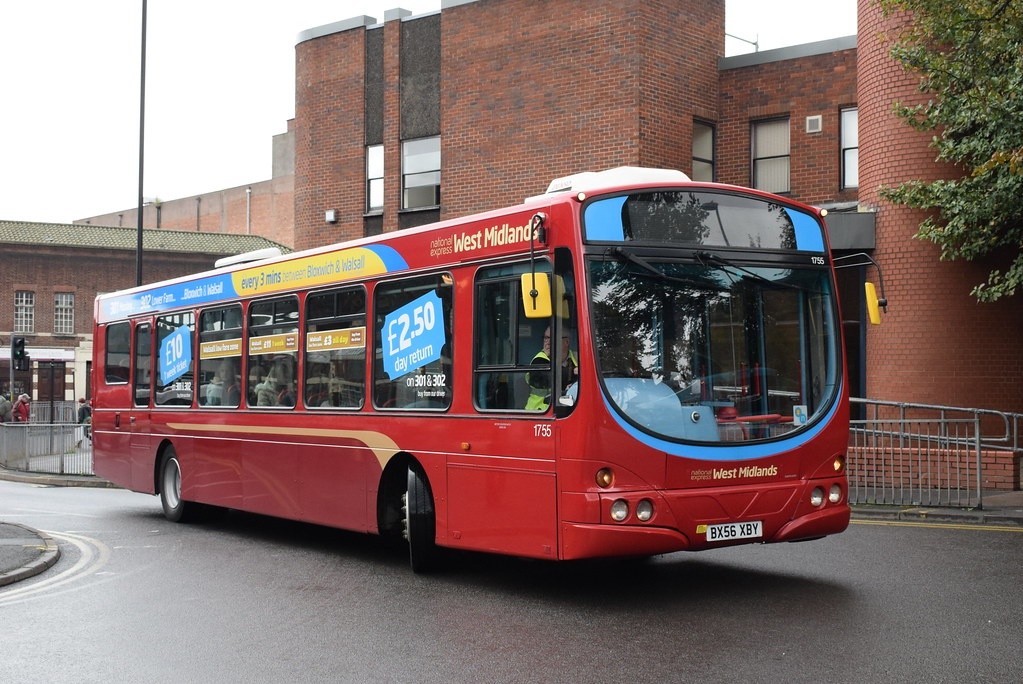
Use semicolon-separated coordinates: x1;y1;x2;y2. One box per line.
11;336;26;359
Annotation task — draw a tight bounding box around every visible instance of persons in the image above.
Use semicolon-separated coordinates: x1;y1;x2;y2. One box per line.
207;324;512;409
525;323;580;412
0;393;31;424
77;398;92;440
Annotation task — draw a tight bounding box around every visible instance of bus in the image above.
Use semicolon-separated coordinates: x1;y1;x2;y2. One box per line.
88;166;890;582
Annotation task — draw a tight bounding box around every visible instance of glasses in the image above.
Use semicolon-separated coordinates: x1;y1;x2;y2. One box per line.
544;336;567;341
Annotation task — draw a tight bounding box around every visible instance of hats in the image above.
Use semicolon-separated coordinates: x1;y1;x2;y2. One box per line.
22;394;30;399
